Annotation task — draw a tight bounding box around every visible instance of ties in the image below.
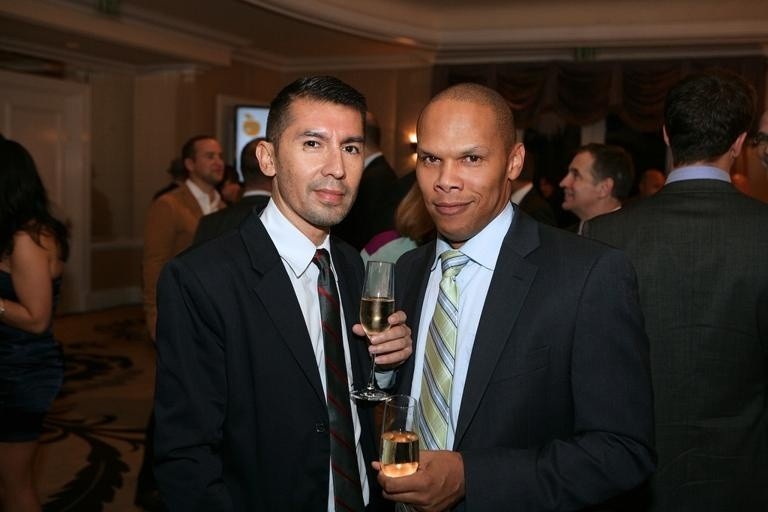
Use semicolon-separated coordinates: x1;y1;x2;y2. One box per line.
313;248;366;511
396;250;470;512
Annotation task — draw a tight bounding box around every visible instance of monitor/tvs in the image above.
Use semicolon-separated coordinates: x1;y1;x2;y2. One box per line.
233;103;270;183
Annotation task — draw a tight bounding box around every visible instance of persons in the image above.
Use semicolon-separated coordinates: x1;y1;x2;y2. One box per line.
140;109;437;343
499;110;768;239
582;74;767;512
0;140;73;512
134;74;414;511
370;82;654;512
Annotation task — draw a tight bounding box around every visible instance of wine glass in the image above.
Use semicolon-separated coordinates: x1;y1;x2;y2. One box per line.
376;396;421;512
348;259;406;401
